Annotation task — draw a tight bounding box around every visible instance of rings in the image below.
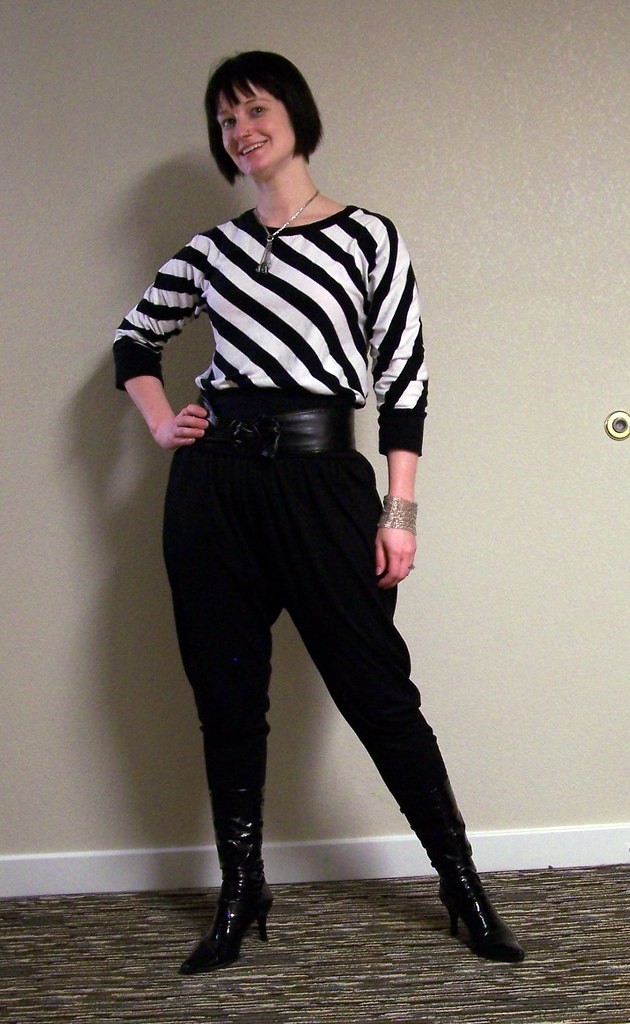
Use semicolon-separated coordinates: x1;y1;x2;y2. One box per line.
409;564;414;569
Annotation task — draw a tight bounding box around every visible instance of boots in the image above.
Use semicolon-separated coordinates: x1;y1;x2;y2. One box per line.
400;776;525;963
178;782;272;974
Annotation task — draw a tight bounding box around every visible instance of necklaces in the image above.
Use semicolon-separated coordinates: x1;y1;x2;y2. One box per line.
254;192;318;272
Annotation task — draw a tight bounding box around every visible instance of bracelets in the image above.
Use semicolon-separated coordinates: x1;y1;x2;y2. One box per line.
378;495;417;534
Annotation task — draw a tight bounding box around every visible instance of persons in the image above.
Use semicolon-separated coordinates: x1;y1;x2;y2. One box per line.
114;51;526;975
